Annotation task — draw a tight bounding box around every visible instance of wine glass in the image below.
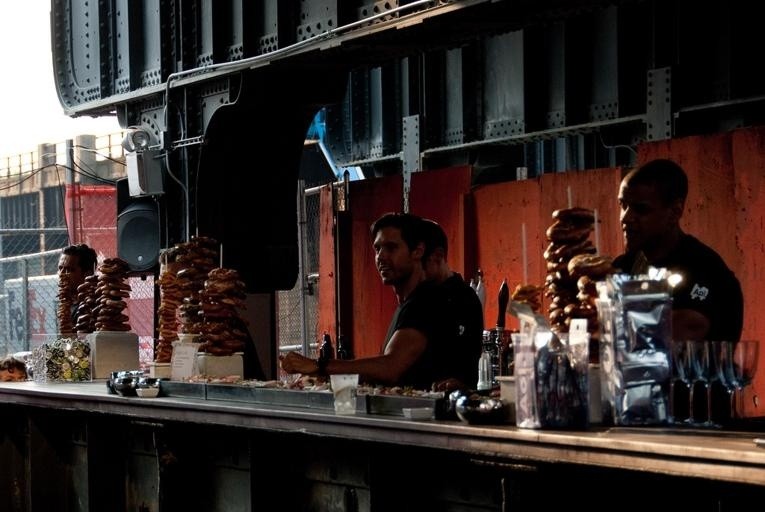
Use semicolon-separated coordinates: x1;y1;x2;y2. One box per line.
671;338;760;427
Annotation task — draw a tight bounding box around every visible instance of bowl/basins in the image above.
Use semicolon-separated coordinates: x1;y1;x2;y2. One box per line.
106;370;161;400
401;406;435;422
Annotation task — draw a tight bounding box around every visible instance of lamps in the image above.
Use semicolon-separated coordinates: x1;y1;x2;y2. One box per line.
122;125;165;197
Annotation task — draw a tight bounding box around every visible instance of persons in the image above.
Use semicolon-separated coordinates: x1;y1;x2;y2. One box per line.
56;243;98;331
1;354;28;382
277;211;462;419
416;214;485;408
611;158;745;428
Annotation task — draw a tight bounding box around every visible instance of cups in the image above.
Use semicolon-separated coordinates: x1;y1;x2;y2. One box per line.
328;373;360;415
